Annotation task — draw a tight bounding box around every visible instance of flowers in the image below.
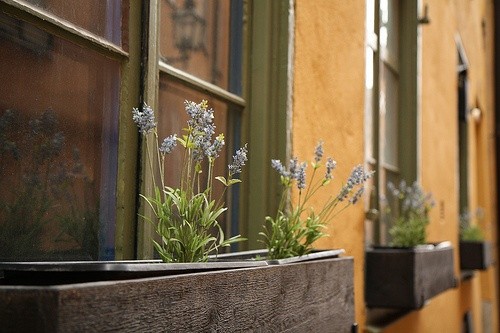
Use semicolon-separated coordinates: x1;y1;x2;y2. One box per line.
380;178;436;250
130;97;251;263
458;204;487;242
254;138;377;262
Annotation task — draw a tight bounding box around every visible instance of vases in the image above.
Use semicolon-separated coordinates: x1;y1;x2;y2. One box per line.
363;240;458;309
0;246;358;333
458;239;494;271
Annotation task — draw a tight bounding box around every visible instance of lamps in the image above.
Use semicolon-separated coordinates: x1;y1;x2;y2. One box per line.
163;0;208;63
